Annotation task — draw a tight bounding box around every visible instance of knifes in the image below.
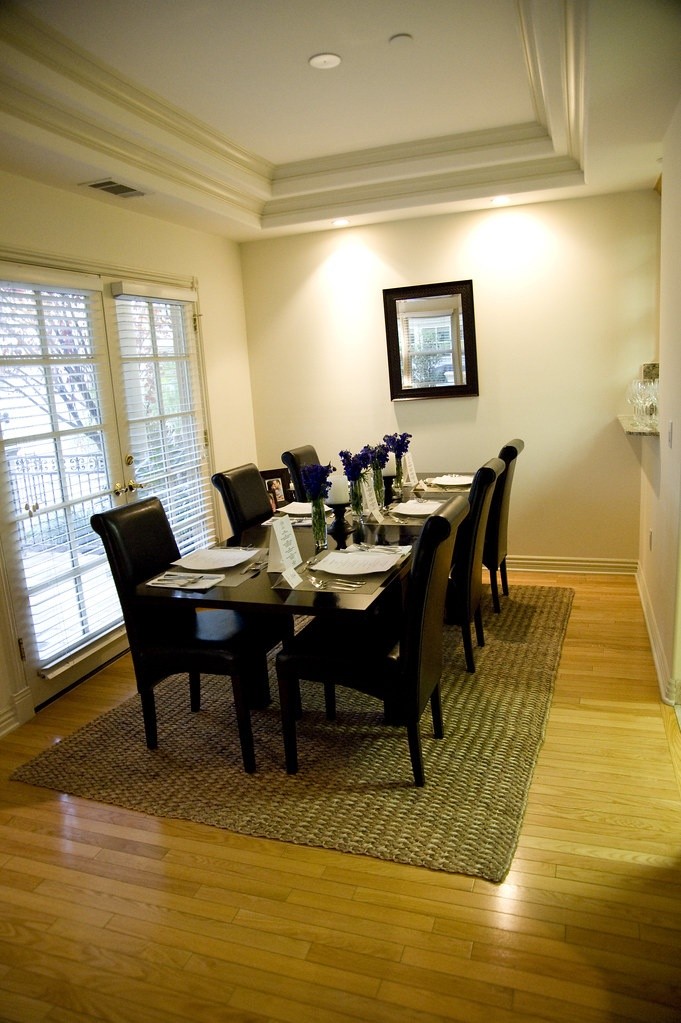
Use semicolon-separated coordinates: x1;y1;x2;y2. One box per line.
164;573;221;580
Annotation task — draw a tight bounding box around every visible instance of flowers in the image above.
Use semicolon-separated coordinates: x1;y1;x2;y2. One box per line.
299;464;336;499
339;449;370;481
382;433;412;455
361;444;389;471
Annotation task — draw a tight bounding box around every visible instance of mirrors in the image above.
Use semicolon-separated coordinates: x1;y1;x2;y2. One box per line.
381;279;478;401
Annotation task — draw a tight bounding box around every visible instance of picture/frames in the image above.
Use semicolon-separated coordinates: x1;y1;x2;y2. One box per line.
266;490;279;515
259;467;292;508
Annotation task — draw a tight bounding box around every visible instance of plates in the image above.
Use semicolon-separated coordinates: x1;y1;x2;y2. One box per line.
169;549;260;570
309;552;403;575
389;502;443;516
431;476;474;486
275;501;333;514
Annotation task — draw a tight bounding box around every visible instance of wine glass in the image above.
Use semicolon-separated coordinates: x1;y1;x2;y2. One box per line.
625;378;659;428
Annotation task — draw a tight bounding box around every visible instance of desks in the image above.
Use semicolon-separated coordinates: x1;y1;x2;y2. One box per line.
137;474;473;774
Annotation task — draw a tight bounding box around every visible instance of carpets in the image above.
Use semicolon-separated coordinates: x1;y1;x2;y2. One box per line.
8;584;574;885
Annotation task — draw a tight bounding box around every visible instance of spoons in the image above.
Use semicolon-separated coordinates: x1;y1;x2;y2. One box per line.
248;562;268;570
297;556;317;574
152;575;204;586
353;542;402;554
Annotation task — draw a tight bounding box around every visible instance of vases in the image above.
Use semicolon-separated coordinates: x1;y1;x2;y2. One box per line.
394;455;403;488
371;464;385;506
311;498;327;547
347;480;364;517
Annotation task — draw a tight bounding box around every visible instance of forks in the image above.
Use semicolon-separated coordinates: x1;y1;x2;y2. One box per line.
305;572;366;587
306;574;366;593
239;555;265;575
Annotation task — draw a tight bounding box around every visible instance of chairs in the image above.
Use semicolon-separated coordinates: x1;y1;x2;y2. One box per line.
89;438;524;788
